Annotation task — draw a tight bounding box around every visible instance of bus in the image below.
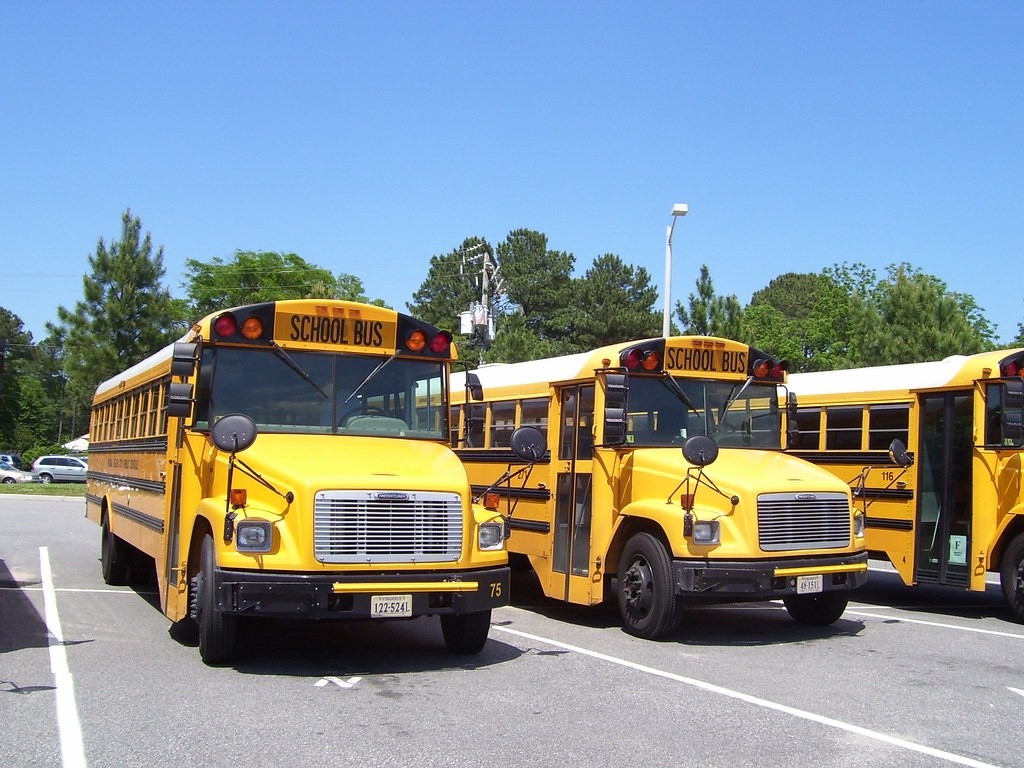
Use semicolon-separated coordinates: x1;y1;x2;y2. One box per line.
573;348;1024;617
82;299;547;667
270;337;914;641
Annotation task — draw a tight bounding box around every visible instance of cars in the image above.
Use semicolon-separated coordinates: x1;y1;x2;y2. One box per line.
0;454;22;470
0;461;41;484
29;456;88;484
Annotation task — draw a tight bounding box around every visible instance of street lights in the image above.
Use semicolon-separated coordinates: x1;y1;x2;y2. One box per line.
664;202;689;339
